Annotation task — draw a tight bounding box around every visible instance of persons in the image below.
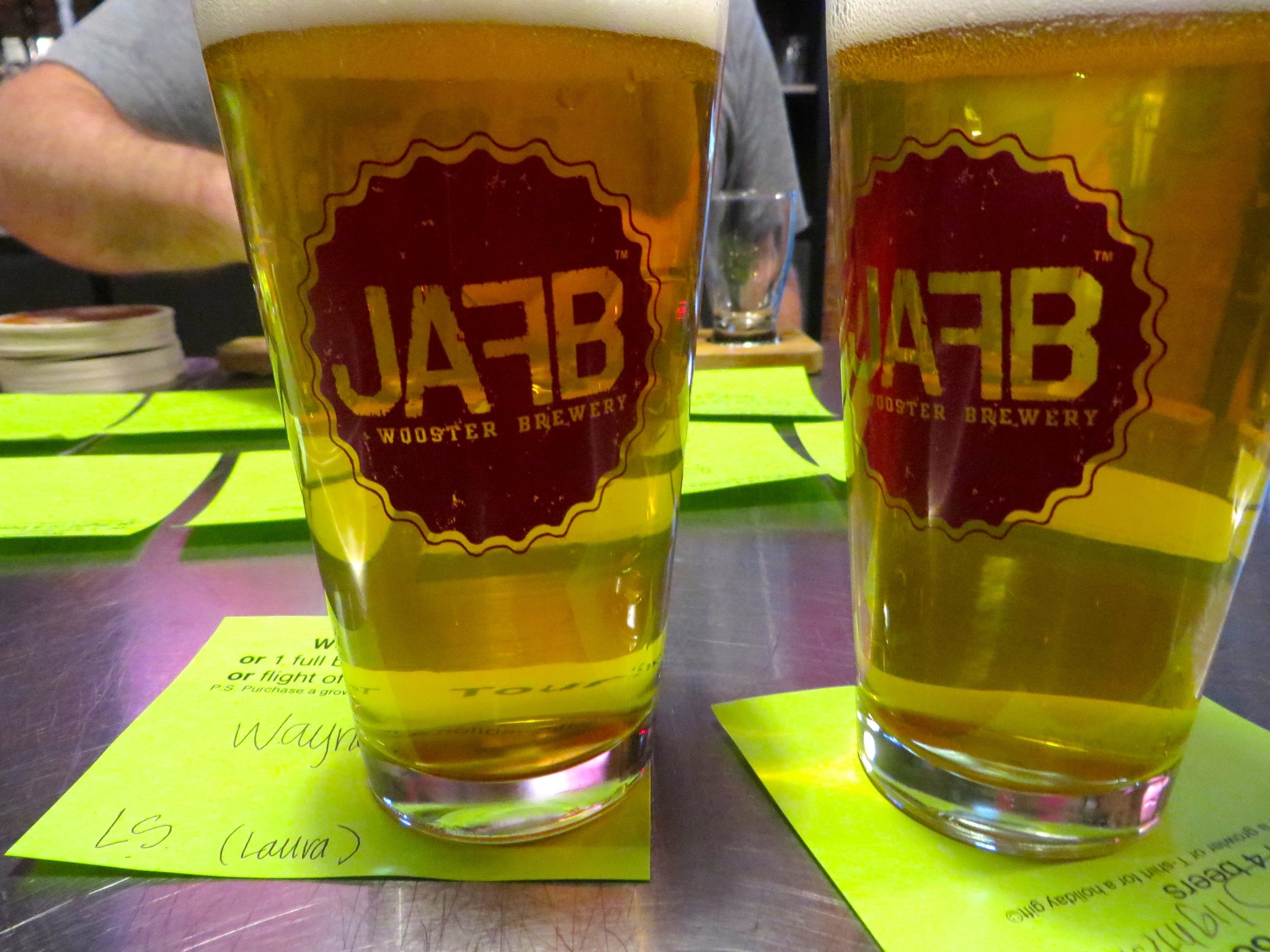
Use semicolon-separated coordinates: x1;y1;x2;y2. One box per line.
0;0;809;356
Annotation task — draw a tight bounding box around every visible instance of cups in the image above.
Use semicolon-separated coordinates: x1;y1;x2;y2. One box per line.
825;0;1270;863
192;0;733;844
771;37;807;87
703;188;800;350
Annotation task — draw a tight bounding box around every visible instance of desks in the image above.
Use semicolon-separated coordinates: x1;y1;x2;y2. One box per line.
0;331;1270;952
